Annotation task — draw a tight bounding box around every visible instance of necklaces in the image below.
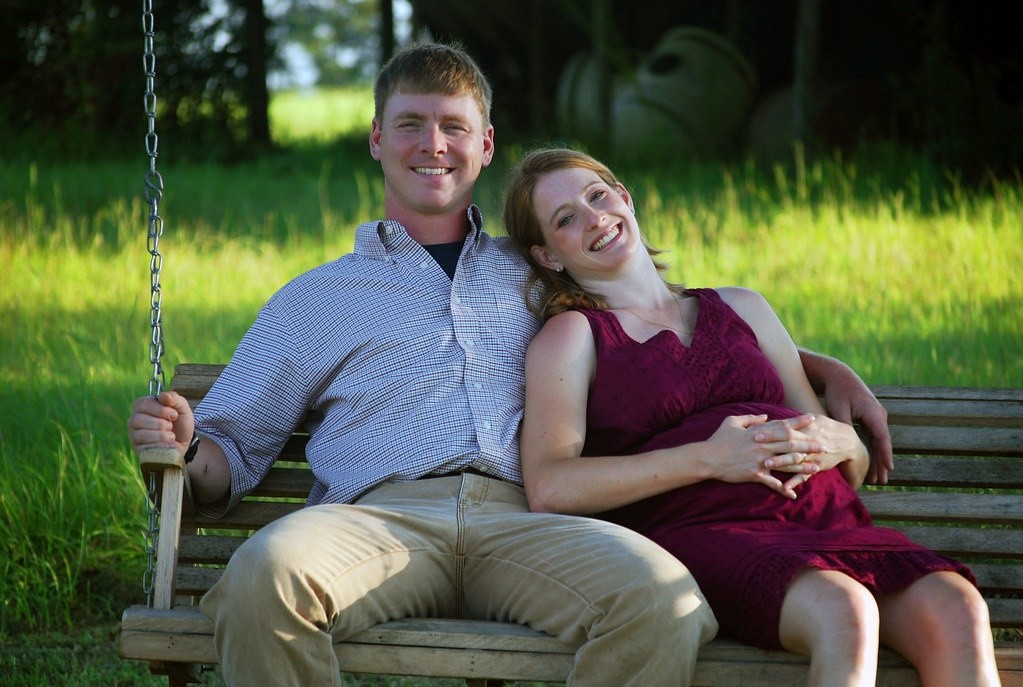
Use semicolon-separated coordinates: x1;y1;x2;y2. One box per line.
625;291;694;340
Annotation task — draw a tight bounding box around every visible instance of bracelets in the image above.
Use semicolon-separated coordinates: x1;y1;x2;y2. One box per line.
184;432;200;463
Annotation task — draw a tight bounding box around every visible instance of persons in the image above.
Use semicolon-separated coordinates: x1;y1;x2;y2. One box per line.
131;44;892;687
504;149;1002;687
